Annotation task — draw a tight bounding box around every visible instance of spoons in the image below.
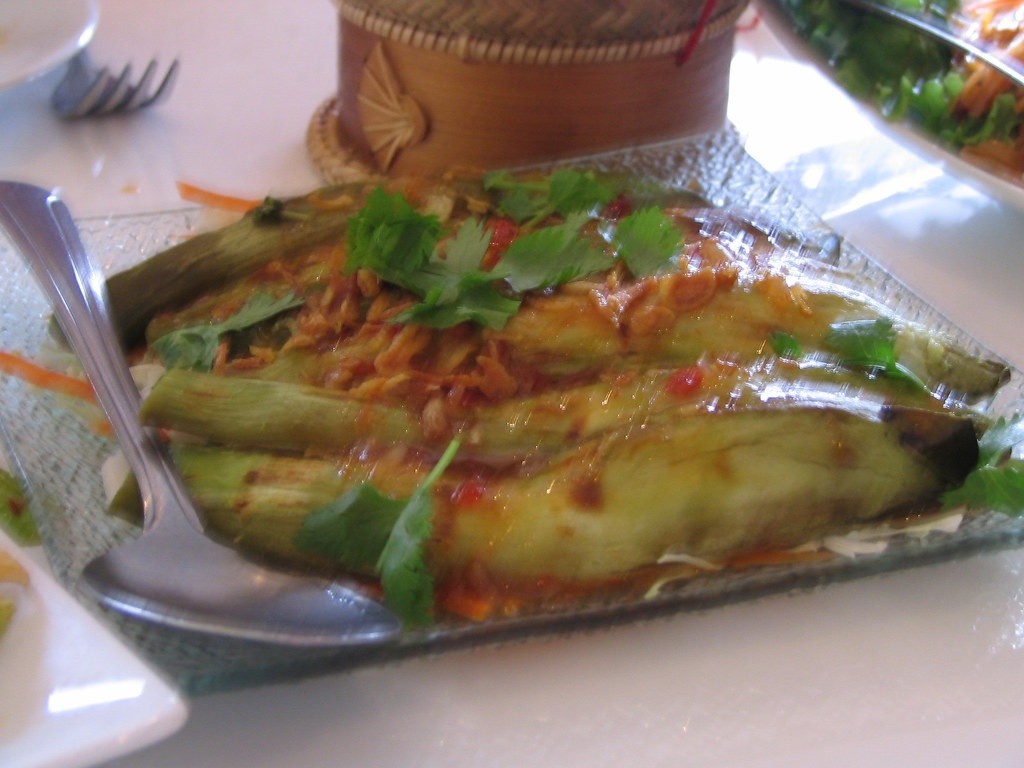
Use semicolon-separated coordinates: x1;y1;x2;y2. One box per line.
0;177;405;656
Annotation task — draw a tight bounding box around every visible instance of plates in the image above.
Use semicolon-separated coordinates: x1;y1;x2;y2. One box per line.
0;1;100;90
0;124;1024;698
746;1;1024;203
0;528;189;767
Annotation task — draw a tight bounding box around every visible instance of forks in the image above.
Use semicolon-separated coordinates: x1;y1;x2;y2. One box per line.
53;52;181;116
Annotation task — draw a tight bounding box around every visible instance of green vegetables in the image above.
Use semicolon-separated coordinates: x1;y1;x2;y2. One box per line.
145;160;1024;638
770;0;1018;151
